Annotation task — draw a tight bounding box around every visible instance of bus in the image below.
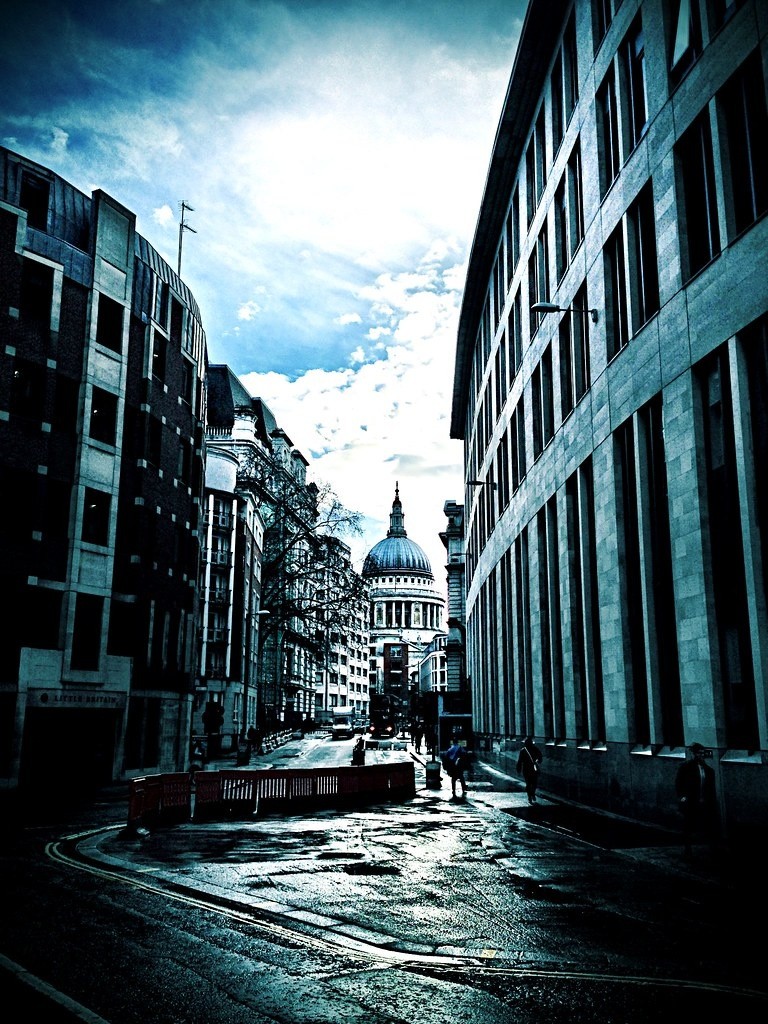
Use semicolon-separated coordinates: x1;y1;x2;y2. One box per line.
369;693;411;737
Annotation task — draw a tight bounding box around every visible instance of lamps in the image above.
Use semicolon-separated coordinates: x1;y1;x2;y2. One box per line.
450;552;472;557
531;303;598;322
467;480;498;491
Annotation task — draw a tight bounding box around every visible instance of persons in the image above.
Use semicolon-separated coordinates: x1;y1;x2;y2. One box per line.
516;739;543;803
188;724;474;799
674;743;717;866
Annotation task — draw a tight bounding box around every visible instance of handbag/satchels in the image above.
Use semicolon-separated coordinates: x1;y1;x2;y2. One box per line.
533;761;542;775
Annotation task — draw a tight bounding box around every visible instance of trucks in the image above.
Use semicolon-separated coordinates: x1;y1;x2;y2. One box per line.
330;705;370;739
